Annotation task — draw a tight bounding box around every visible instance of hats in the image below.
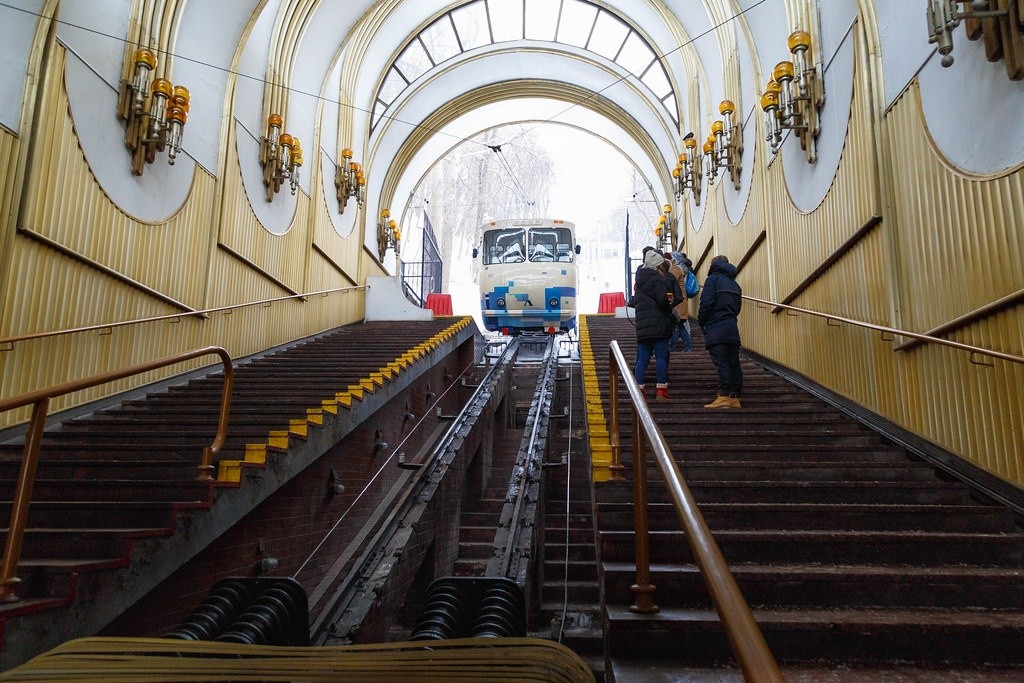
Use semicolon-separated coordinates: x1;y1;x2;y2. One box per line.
642;246;663;263
643;249;664;269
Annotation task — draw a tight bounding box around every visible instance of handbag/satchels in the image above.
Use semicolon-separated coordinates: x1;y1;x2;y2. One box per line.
683;272;700;300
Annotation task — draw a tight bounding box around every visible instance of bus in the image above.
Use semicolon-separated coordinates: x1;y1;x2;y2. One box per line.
472;218;582;335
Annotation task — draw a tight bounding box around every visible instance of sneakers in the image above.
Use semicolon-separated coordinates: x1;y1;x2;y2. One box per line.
639;384;648;399
704;395;731;409
730;396;742;410
656;383;673;400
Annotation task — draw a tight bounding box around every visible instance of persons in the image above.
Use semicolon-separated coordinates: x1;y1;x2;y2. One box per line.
698;255;744;409
633;246;700;399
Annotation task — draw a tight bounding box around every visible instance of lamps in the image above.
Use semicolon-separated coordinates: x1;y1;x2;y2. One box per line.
341;148;365;210
656;205;674;252
129;49;190;165
673;135;696;202
381;209;401;259
703;99;739;185
268;113;304;195
761;25;816;153
